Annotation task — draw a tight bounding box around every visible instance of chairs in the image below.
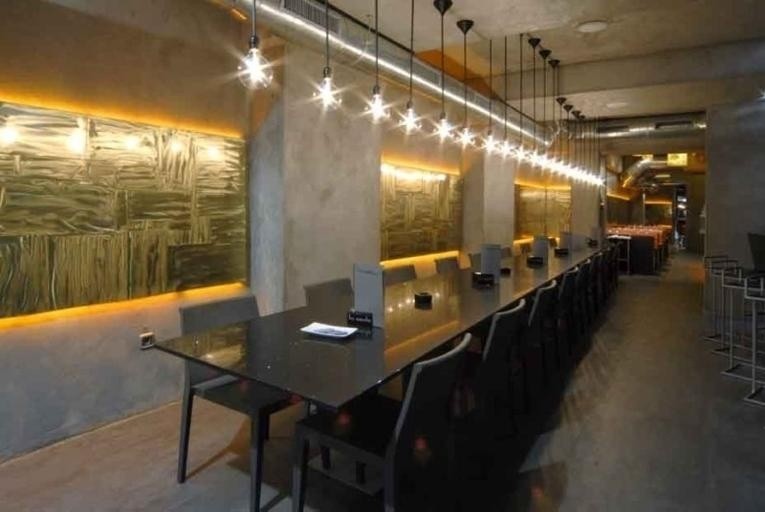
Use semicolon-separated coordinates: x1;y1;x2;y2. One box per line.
288;334;472;511
522;243;615;383
433;255;458;273
469;232;571;268
301;277;382;418
605;222;672;277
177;294;329;512
382;265;416;285
458;297;526;427
702;252;764;407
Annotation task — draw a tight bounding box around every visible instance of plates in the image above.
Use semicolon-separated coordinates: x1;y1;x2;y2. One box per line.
298;321;359;340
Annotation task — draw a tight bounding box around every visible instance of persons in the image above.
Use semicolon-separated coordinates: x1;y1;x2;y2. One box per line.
676;214;686;247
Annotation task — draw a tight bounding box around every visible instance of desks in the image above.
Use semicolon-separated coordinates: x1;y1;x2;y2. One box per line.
152;247;617;512
